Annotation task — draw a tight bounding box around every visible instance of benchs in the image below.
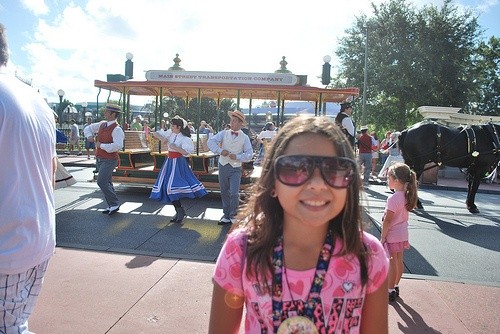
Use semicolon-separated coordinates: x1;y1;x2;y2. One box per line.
117;131;219;174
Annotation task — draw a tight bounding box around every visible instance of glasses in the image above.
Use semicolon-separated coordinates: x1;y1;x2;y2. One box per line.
273;154;358;188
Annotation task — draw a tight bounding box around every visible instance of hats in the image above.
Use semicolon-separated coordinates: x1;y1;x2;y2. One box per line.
340;103;354;108
227;109;248;126
105;103;125;113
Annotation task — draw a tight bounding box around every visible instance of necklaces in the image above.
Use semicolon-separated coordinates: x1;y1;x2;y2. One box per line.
272;221;336;333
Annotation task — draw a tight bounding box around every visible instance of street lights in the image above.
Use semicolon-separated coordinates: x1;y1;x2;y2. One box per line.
81;102;88;139
123;51;135;123
321;53;332;118
57;89;65;127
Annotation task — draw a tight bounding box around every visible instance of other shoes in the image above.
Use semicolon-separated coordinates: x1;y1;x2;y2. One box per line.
64;152;70;155
88;158;90;160
388;286;399;301
102;207;120;215
77;153;83;156
218;217;236;225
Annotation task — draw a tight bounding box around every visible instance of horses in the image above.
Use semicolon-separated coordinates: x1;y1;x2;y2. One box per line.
398;119;500;214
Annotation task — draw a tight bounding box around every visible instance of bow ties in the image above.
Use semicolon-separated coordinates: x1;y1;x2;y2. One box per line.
231;131;238;136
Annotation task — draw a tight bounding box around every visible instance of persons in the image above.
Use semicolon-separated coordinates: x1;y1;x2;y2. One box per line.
69;117;99;160
379;163;418;303
145;117;209;224
83;104;125;216
0;24;57;334
335;103;405;189
208;115;389;334
123;114;280;169
207;110;254;225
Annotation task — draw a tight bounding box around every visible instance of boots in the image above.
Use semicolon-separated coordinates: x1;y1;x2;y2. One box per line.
170;206;186;223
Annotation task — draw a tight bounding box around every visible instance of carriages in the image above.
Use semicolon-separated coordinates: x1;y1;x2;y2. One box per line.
92;52;500;211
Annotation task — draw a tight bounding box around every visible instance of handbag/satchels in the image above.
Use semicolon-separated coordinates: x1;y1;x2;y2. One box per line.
379;149;389;154
